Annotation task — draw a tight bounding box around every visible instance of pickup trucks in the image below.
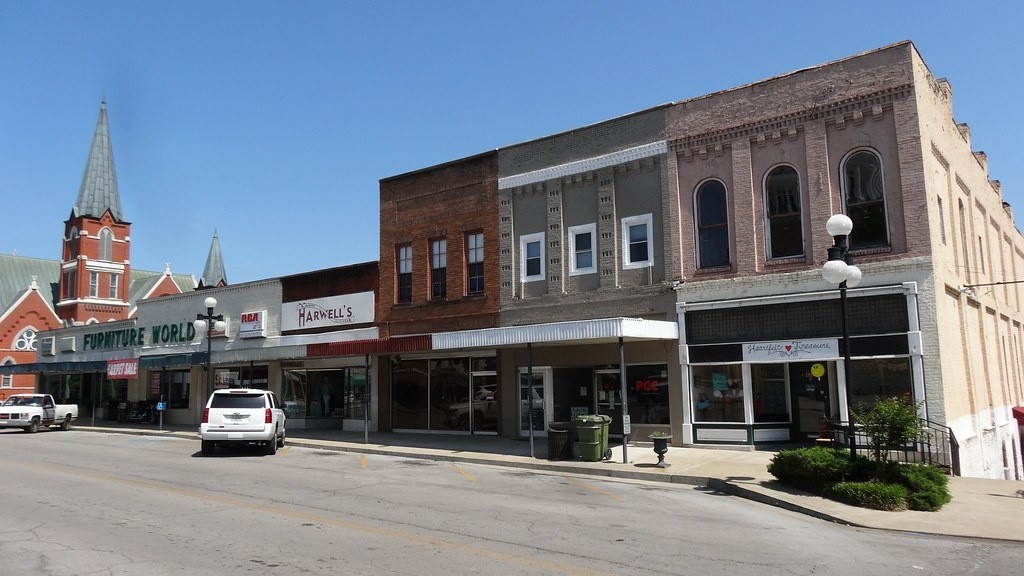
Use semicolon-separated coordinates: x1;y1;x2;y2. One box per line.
0;393;79;434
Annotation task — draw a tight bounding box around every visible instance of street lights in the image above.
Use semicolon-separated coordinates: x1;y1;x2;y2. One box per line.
821;211;863;458
194;295;228;401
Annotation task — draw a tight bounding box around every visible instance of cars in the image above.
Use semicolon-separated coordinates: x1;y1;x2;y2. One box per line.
199;387;286;453
446;382;544;431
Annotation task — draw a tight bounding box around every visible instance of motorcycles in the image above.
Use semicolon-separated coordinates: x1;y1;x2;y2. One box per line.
126;399;159;424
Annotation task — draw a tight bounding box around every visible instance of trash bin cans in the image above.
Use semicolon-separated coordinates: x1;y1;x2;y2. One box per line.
547;421;578;462
575;413;613;461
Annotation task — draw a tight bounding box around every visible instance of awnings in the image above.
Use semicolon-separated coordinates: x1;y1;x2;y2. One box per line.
0;362;108;376
139;352;207;368
207;316;681;464
